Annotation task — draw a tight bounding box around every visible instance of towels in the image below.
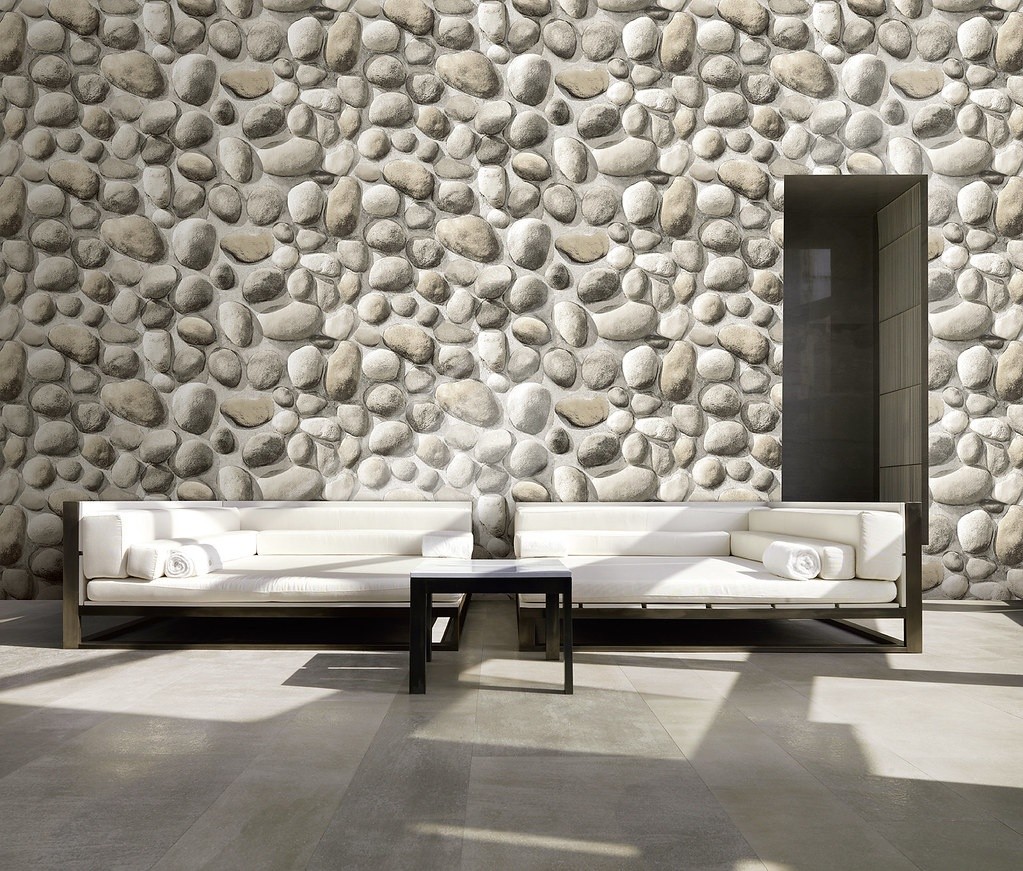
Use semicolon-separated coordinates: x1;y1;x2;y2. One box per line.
761;540;822;581
163;542;224;580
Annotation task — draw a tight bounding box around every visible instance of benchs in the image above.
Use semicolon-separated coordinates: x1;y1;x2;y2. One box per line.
512;500;923;656
61;498;475;652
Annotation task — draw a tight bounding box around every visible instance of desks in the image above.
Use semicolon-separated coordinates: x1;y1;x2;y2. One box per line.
408;559;574;695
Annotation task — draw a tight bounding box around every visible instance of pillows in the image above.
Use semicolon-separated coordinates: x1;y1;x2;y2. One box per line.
80;507;241;578
254;529;435;556
513;505;750;532
237;507;472;531
730;529;858;580
421;530;475;558
748;508;904;582
513;530;730;558
126;530;258;581
